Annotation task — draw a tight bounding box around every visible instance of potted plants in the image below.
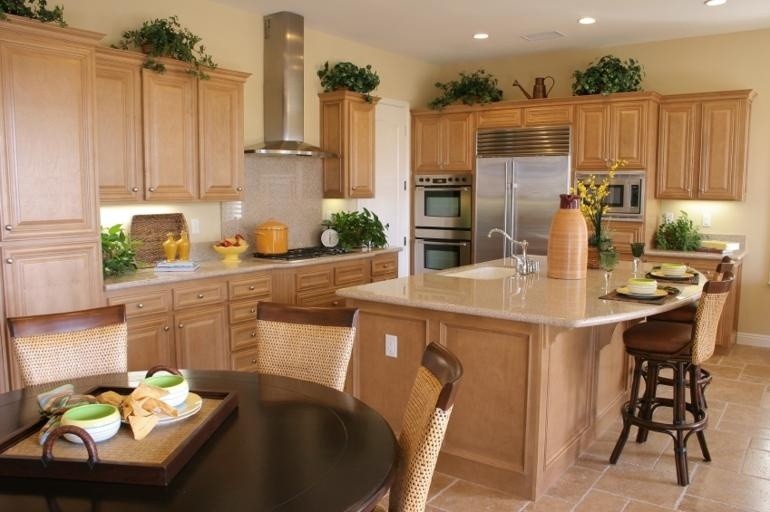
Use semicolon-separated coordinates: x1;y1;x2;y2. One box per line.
425;66;503;113
654;209;702;251
113;14;218;81
317;62;381;104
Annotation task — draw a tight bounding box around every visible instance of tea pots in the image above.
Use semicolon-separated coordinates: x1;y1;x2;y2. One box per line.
547;194;588;280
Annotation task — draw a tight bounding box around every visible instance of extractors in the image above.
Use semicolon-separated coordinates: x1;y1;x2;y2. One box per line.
244;11;341;159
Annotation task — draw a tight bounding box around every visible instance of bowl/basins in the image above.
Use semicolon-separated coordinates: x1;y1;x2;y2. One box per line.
627;277;658;294
662;263;686;276
143;375;188;408
60;404;120;444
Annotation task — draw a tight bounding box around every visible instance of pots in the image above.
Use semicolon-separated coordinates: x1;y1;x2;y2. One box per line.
253;219;289;254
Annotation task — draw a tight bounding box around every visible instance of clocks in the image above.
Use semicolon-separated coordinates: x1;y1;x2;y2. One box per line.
319;224;341;254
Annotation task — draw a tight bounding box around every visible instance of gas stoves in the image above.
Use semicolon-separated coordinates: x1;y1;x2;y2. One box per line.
252;247;351;263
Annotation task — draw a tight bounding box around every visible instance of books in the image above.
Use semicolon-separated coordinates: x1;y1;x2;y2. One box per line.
154;262;200;272
696;240;739;254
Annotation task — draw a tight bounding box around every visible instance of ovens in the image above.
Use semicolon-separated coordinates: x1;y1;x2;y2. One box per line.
412;171;474;274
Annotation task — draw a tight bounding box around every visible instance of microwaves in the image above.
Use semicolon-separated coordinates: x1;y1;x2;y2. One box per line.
576;173;644;218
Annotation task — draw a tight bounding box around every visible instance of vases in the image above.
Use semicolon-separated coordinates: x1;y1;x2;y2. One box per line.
586;240;608;270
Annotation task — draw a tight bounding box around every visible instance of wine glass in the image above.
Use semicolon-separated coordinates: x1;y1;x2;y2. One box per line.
630;242;646;275
599;249;617;296
214;245;250;264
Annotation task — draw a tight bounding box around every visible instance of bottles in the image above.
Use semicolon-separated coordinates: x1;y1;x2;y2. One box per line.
162;232;177;261
177;231;190;262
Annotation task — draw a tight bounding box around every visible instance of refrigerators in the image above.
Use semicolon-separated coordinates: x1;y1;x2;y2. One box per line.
474;125;572;264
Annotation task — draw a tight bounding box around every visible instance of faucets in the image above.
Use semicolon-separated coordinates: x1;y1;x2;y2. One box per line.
487;228;527;276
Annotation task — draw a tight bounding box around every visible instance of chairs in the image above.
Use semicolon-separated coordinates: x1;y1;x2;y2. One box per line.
360;341;462;512
638;255;733;417
609;271;735;487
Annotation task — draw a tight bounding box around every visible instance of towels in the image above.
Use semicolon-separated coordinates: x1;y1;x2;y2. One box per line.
96;387;179;441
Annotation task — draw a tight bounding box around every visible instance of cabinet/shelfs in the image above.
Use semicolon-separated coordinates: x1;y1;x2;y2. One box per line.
0;13;106;240
93;45;196;205
197;63;252;204
584;217;663;271
317;90;375;199
645;253;742;356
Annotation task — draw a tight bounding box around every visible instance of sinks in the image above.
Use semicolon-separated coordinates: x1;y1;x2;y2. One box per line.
438;267;517;281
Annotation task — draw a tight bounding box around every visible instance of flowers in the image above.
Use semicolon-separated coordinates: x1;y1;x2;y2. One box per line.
571;159;628;246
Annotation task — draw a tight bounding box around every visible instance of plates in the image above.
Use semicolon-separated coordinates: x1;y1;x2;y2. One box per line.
650;270;697;280
120;392;203;426
616;288;669;299
